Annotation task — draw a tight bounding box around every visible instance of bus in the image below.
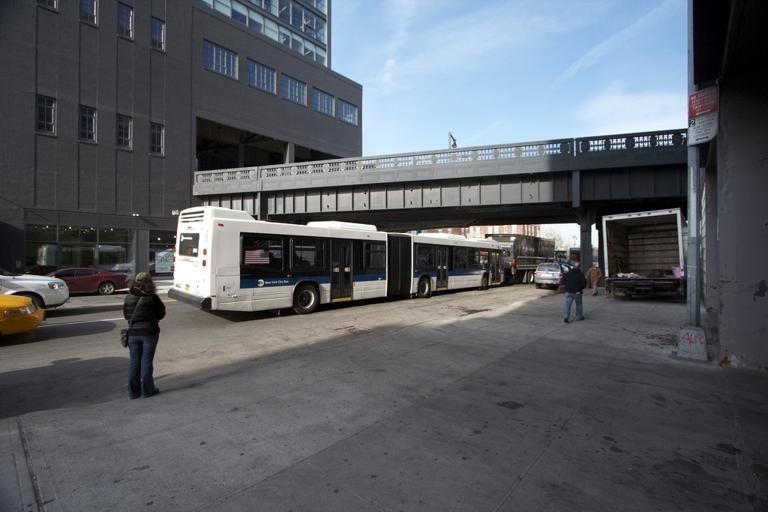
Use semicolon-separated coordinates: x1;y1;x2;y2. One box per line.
167;205;508;315
38;244;173;278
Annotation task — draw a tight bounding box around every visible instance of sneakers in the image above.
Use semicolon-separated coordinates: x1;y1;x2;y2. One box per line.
128;387;159;399
564;317;569;323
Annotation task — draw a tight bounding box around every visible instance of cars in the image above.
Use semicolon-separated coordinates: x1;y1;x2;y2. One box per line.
0;264;131;337
532;262;568;290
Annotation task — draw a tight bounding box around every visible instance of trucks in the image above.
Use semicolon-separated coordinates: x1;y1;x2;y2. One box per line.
484;233;567;286
600;207;687;301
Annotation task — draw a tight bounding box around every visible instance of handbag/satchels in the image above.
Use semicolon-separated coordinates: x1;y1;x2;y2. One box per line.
119;328;129;348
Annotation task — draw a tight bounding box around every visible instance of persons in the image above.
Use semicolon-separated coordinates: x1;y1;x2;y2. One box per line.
123;271;167;400
585;261;603;297
560;261;585;323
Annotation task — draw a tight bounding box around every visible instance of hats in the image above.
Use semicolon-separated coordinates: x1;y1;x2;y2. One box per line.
136;272;151;282
